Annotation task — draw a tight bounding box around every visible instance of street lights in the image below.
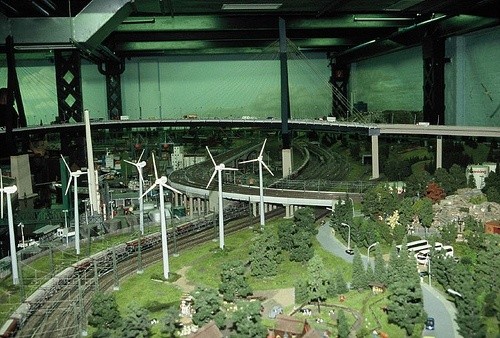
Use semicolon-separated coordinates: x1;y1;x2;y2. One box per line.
82;199;89;225
342;222;351;250
327;207;335;213
448;288;464;300
348;198;354;219
17;223;25;251
426;256;432;288
367;241;380;265
63;210;69;248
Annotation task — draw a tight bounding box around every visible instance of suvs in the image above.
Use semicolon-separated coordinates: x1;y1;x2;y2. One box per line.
346;249;353;255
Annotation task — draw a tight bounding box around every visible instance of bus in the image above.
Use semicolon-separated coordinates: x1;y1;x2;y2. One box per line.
408;242;443;261
397;239;428;258
416;246;454;264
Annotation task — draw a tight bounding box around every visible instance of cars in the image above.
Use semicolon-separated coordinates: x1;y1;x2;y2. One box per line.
426;318;435;331
